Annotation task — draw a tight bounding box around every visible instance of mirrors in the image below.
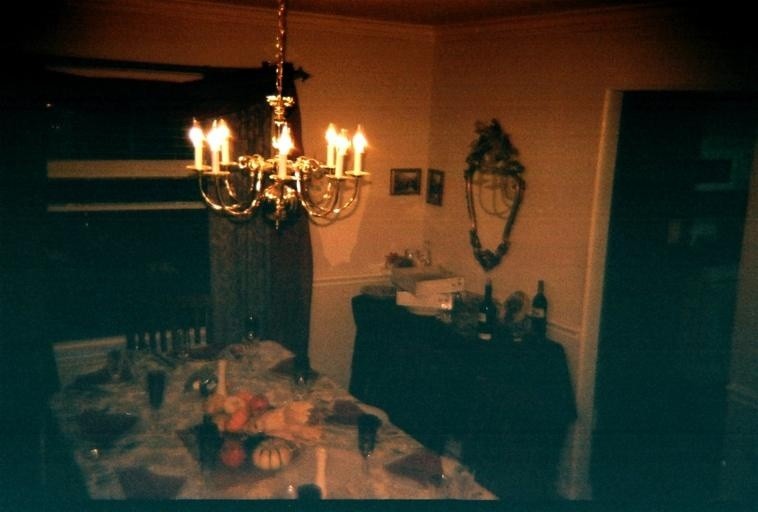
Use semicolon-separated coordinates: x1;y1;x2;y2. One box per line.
462;117;524;275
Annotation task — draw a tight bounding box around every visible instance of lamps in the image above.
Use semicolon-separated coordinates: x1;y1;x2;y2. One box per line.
187;1;373;229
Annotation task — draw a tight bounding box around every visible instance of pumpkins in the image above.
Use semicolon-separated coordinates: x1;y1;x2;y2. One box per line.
220;439;244;467
207;388;269;432
253;438;291;471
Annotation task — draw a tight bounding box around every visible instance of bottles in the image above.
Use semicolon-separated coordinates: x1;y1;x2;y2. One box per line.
531;281;548;335
477;278;496;342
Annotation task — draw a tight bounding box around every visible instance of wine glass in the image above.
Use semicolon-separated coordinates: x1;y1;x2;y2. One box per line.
145;370;166;434
357;412;382;465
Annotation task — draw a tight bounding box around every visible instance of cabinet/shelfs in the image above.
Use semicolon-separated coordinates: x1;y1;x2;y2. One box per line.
350;296;568;499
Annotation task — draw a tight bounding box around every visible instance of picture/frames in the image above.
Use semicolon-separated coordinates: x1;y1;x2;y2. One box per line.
389;167;444;206
